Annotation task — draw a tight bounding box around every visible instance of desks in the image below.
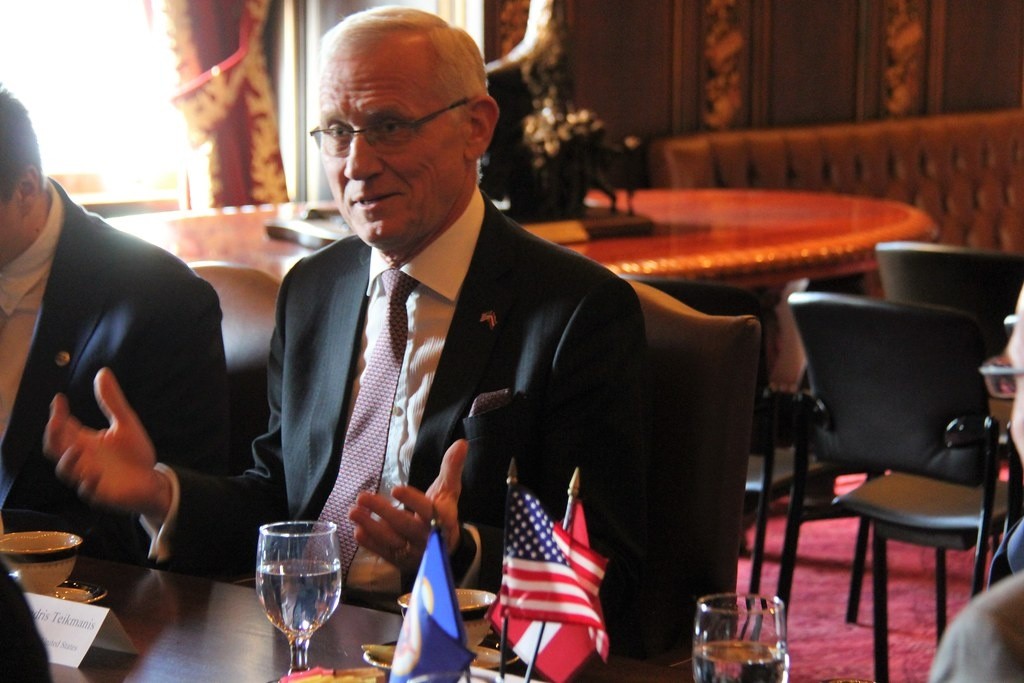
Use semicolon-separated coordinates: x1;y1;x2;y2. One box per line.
44;557;693;683
94;186;938;555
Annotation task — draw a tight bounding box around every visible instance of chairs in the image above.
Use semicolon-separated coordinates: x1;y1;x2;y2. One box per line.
604;238;1024;683
190;265;282;473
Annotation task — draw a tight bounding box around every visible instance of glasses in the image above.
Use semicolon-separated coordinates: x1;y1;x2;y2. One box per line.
979;355;1024;399
310;95;476;156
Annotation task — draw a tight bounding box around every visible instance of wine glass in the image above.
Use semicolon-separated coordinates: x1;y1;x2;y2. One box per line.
255;520;342;683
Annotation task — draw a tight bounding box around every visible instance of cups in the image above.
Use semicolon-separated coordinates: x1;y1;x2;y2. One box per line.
1;531;85;595
691;591;790;683
397;588;497;649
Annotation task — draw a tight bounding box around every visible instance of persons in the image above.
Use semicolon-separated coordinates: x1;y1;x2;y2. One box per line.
922;277;1024;683
41;6;648;609
0;81;232;566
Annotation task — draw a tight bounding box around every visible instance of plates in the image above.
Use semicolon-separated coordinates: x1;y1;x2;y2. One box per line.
48;579;109;604
363;636;519;670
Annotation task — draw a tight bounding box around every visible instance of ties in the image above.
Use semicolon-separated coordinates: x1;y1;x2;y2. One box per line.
302;268;419;603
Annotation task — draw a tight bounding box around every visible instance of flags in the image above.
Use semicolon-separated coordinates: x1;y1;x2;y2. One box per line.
484;500;602;683
498;486;610;662
388;528;475;683
